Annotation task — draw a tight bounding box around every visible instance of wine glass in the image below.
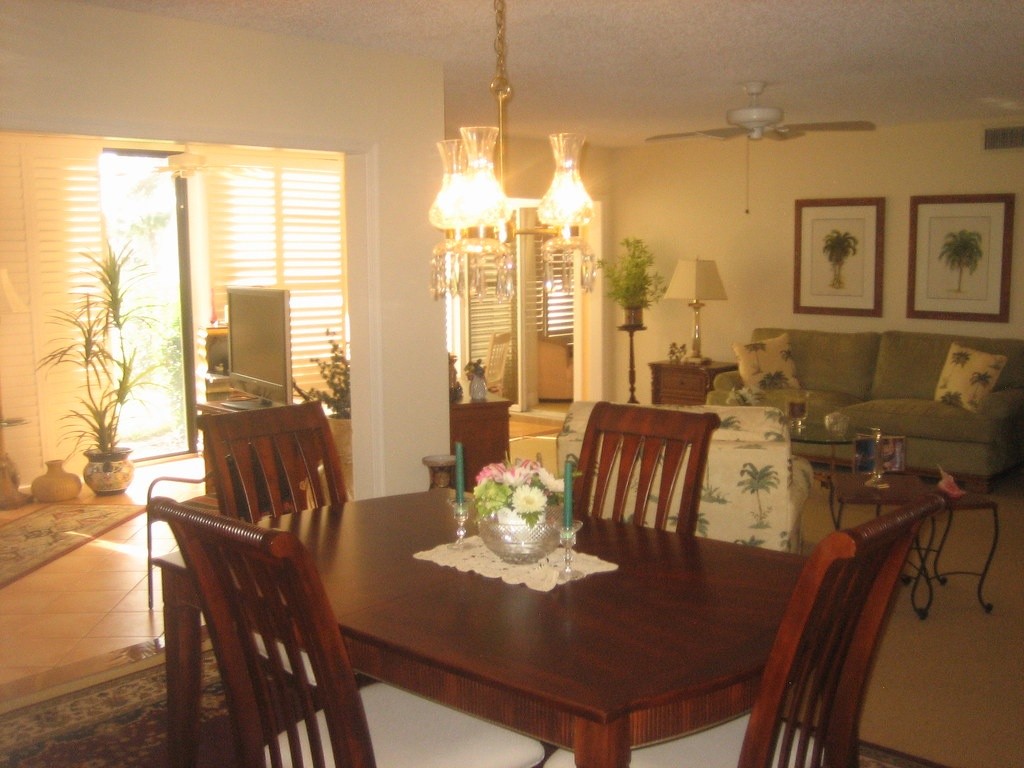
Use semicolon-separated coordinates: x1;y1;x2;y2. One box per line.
782;390;808;435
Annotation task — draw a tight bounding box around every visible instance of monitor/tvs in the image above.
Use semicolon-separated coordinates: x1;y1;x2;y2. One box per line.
221;287;292;410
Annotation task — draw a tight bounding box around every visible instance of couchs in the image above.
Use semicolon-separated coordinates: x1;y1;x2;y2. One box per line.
539;334;573;399
555;328;1024;553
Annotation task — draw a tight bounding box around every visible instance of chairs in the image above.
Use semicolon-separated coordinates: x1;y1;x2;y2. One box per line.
464;332;511;402
144;403;953;768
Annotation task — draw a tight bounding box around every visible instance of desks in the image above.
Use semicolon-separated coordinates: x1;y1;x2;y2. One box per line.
192;398;296;493
147;489;809;768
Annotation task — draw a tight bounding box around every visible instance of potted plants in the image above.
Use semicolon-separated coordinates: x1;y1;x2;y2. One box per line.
598;238;666;327
27;238;170;495
295;329;352;461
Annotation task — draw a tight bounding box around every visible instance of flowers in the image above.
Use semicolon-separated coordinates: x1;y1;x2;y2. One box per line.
464;358;486;381
471;449;565;527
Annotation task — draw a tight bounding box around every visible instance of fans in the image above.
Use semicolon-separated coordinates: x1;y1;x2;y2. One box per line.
153;142;242;180
644;81;876;143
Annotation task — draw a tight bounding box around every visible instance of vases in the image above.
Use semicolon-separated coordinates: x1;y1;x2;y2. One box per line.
32;459;82;502
477;506;564;563
449;359;485;400
423;453;456;490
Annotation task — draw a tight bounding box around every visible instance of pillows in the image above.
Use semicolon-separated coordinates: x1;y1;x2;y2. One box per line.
731;332;1007;411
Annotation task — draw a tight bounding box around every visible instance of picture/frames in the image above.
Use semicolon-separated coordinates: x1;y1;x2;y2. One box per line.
794;194;1015;322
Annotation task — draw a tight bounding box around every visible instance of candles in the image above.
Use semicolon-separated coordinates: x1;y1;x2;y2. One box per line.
563;462;573;529
455;441;465;506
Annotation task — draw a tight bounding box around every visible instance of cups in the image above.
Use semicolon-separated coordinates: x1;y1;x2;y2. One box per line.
825;416;849;437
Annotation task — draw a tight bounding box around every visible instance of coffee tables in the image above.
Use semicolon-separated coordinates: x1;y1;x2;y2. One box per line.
788;422;999;618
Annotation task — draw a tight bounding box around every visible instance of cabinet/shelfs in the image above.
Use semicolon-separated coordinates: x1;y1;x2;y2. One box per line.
648;357;738;405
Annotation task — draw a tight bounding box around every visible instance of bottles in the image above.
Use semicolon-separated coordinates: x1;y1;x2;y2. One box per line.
31;459;82;503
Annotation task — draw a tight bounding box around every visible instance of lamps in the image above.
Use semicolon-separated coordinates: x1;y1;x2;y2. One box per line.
663;256;727;364
429;1;601;301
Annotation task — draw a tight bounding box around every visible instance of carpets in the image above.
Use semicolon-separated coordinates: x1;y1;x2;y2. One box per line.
0;502;959;768
